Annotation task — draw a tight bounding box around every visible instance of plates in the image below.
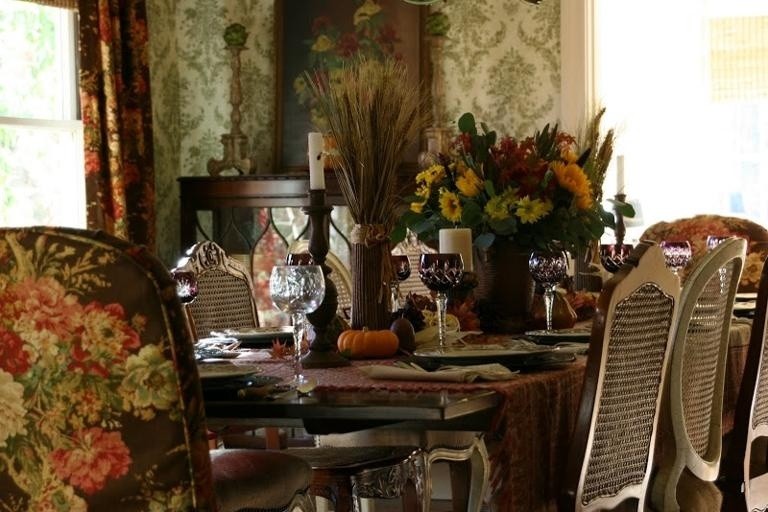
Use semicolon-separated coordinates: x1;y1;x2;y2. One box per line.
732;299;754;315
197;363;262;385
733;293;760;301
521;327;591;343
410;346;580;374
217;322;297;344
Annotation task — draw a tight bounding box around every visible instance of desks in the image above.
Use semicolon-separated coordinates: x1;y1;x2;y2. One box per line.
173;176;421;257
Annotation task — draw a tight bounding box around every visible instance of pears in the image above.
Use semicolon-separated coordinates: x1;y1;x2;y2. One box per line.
390;313;415;348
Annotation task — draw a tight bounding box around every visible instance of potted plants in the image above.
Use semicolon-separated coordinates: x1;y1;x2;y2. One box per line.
293;48;444;330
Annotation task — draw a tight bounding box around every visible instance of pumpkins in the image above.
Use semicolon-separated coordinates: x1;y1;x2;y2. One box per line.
337;327;398;359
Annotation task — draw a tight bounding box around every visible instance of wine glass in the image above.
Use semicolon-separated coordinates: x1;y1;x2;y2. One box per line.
526;243;571;342
284;239;316;266
600;234;735;297
269;264;323;393
391;253;411;314
414;250;465;355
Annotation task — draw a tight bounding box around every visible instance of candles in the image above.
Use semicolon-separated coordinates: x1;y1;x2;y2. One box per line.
616;155;625;195
307;132;324;191
439;229;474;273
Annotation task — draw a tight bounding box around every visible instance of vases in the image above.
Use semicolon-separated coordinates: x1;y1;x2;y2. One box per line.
462;244;537;333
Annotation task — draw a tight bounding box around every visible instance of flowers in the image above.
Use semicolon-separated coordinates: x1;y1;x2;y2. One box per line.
386;103;635;266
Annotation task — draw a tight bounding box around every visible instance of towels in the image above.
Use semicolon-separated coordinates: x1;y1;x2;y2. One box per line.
355;362;524;383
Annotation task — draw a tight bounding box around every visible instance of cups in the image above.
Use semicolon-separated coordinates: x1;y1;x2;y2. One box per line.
169;268;198;306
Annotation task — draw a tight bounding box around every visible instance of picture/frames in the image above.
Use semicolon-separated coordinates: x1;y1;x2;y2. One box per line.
273;0;426;174
